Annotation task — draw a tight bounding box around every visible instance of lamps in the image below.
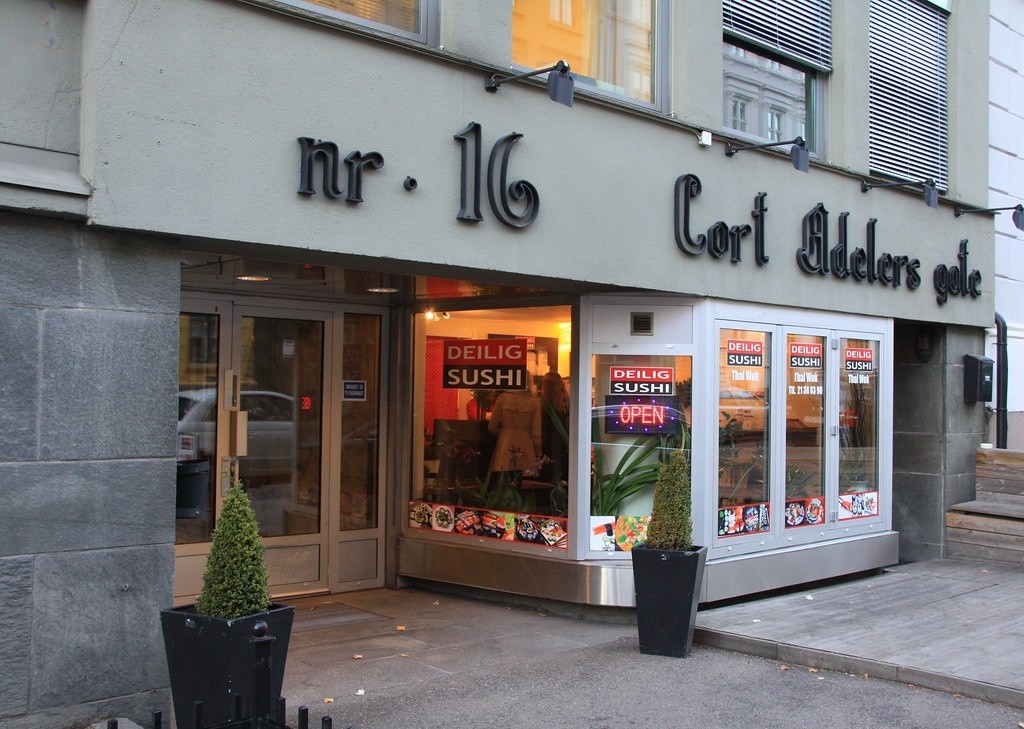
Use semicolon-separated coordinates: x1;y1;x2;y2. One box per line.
723;135;810;174
954;204;1024;233
859;177;940;209
604;394;680;435
483;60;576;109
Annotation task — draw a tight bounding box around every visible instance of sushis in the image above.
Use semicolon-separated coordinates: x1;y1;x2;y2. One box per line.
592;522;615;536
408;501;566;546
718;493;877;536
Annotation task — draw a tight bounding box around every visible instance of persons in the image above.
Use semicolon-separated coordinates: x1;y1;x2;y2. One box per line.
484;369;543;501
466;389;495;421
240;401;268;421
536;371;570;506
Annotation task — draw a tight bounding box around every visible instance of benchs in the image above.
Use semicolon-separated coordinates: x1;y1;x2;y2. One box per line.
942;445;1024;568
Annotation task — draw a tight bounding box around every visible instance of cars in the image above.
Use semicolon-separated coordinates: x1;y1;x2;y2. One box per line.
178;388;298;482
718;385;765;432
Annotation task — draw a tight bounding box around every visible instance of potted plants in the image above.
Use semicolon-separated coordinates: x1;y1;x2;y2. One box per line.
631;448;709;658
158;473;298;729
